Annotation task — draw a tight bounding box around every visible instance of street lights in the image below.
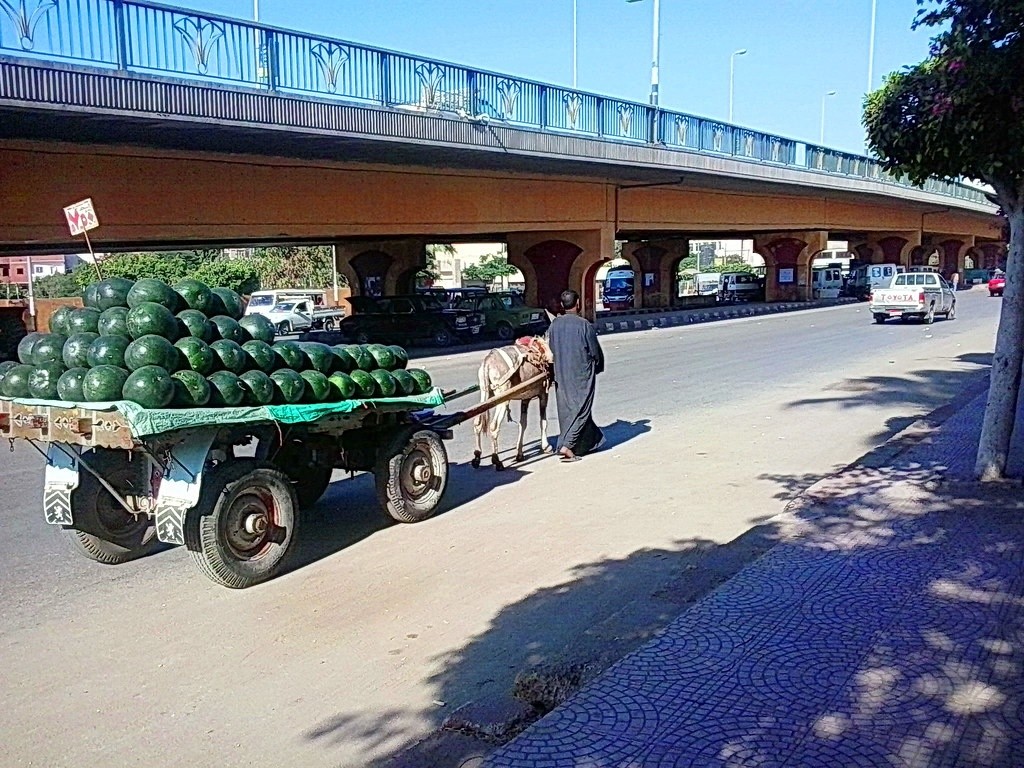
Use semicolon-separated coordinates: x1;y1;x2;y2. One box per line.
820;91;836;145
629;0;659;106
730;49;747;123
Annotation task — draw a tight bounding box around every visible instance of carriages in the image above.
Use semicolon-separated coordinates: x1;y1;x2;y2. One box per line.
0;312;558;589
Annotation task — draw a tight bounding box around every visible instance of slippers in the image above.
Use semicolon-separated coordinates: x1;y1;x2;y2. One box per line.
560;456;580;462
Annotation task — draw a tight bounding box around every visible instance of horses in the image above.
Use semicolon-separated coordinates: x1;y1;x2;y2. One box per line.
472;308;557;471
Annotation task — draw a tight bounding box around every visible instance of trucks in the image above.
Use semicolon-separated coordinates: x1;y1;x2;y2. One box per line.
693;272;721;296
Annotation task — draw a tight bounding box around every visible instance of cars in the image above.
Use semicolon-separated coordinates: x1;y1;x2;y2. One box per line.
456;292;547;339
340;295;486;347
989;273;1006;296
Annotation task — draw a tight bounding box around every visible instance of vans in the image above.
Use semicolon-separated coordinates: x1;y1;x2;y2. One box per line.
813;265;844;298
856;263;898;299
244;290;327;319
718;271;765;299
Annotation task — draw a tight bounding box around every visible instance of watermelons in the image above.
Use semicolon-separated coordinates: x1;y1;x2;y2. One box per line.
0;277;433;408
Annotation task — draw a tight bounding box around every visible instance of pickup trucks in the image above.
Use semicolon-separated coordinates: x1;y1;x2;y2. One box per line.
261;299;346;331
868;271;956;324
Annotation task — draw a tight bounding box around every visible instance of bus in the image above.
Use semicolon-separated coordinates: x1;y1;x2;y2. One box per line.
601;265;654;311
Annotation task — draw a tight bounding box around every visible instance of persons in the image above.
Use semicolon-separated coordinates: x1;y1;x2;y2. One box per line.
950;270;959;293
545;289;604;462
317;297;324;305
941;269;949;280
